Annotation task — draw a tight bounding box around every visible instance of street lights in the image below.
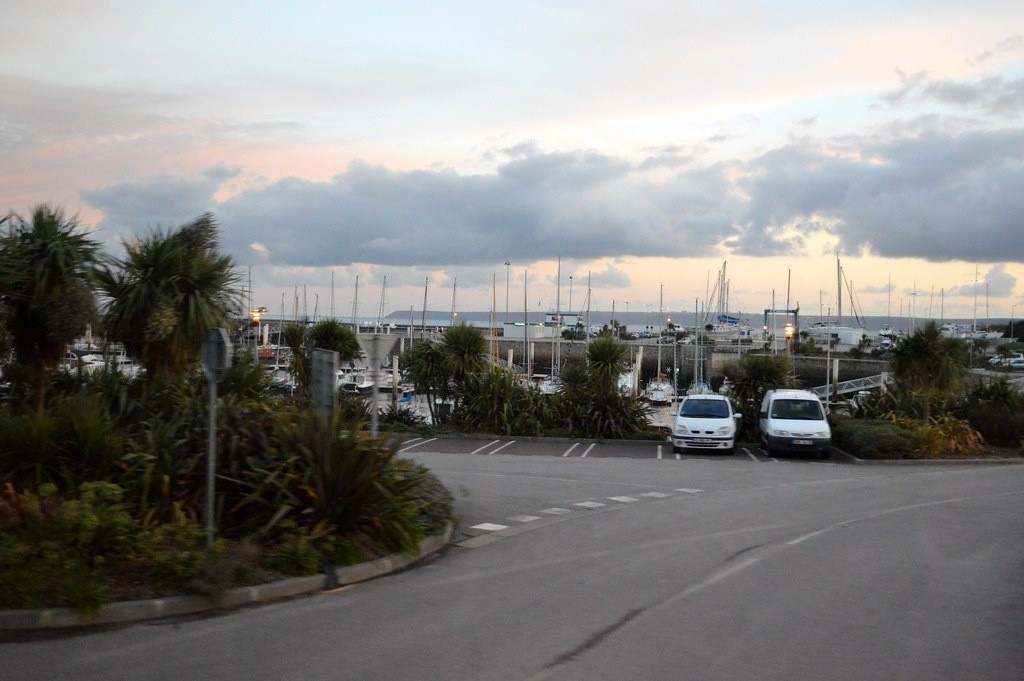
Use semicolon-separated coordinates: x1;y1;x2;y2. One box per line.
504;260;511;323
784;323;794;386
252;309;261;368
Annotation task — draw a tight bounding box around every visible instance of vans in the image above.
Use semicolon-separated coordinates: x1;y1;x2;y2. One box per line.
989;353;1024;366
761;387;832;459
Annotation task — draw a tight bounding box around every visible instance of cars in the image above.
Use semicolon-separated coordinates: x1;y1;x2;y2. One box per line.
669;394;741;456
1002;359;1024;369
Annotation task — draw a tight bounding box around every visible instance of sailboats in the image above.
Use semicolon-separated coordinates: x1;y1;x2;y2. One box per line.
212;259;1023;425
